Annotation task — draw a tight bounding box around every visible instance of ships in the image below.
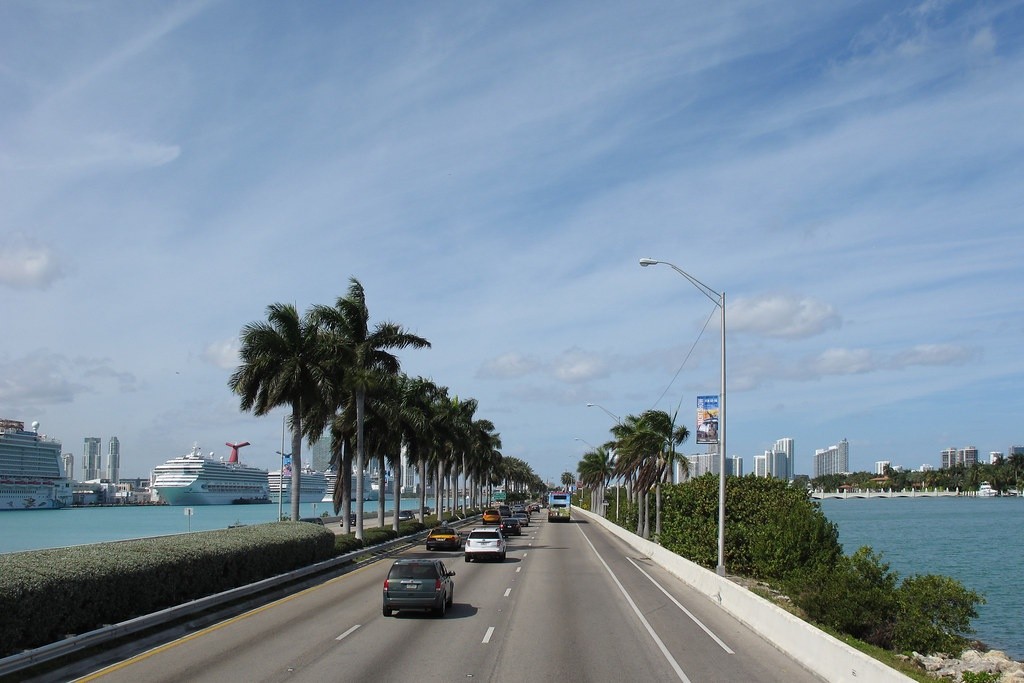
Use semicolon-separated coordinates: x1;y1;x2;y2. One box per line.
325;462;373;503
149;441;271;507
268;450;330;504
369;467;395;501
0;420;74;510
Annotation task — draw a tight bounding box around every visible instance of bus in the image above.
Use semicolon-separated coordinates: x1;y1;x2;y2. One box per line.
547;491;572;523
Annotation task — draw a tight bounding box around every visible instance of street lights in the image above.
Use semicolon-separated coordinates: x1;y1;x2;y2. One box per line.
587;402;621;520
639;258;729;579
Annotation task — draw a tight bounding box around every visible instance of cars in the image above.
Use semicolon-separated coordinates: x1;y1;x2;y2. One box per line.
482;509;502;525
340;513;356;527
299;517;324;526
498;505;511;517
399;510;415;521
418;506;431;516
500;518;521;536
382;558;456;619
425;527;463;552
512;503;544;527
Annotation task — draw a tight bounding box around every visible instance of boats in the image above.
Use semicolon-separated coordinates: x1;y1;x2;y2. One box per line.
1;480;14;484
15;480;27;484
28;481;41;485
43;481;55;485
979;480;998;497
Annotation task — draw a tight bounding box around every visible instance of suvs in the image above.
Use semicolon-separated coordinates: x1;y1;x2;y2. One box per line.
465;525;507;562
